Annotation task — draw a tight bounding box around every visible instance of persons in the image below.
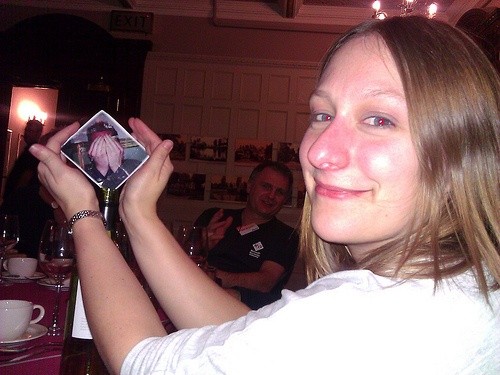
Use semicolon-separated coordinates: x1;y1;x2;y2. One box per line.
31;16;500;375
186;162;299;313
7;120;43;215
84;120;141;188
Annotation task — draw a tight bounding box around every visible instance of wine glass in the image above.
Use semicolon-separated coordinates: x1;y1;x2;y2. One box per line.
0;214;20;271
38;219;77;346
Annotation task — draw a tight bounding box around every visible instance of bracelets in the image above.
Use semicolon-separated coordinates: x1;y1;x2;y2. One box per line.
66;210;107;235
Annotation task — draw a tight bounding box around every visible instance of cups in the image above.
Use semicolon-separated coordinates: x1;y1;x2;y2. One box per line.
0;300;45;341
3;257;37;277
177;224;210;276
109;209;135;274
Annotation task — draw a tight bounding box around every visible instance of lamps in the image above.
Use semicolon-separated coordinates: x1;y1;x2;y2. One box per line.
371;0;438;20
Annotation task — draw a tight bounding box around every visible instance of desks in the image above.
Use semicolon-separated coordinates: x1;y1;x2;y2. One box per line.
0;269;241;375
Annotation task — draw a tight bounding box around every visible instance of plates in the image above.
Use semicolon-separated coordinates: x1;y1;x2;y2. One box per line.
36;278;70;289
0;271;46;280
0;324;49;344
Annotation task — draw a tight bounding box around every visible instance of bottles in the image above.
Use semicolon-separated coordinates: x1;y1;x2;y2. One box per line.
58;186;119;375
